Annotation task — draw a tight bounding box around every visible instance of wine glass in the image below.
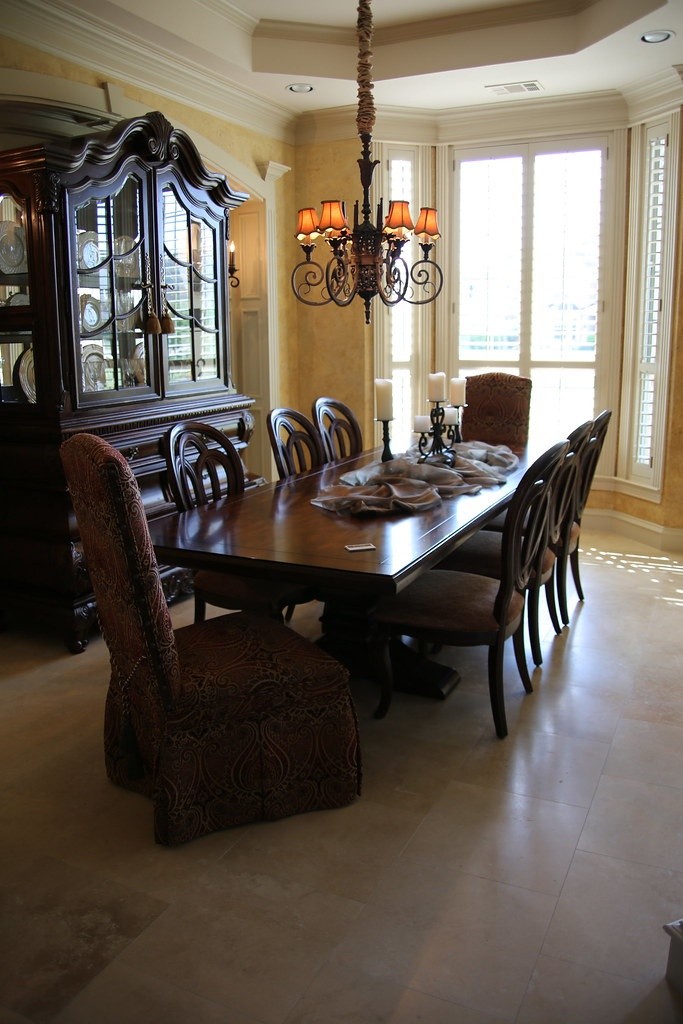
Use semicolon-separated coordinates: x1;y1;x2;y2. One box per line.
118;293;134;333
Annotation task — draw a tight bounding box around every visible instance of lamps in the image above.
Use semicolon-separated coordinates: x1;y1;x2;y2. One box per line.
291;0;444;324
228;240;241;287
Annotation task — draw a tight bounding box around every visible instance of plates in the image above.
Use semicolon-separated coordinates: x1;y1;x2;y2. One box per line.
10;294;32;335
19;347;36;403
82;344;112;391
78;231;103;275
0;220;28;274
114;236;138;278
81;297;103;332
133;342;147;384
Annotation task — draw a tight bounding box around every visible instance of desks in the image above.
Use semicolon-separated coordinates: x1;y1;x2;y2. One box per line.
146;439;554;701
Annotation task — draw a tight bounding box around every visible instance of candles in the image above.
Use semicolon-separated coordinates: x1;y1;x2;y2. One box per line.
374;377;392;420
414;416;430;432
429;372;445;401
444;408;457;424
450;378;467;405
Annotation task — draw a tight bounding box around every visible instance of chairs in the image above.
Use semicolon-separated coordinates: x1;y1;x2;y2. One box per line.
312;396;362;463
430;423;595;667
368;439;571;739
461;373;532;453
267;406;325;479
163;421;246;622
482;411;612;626
59;433;363;846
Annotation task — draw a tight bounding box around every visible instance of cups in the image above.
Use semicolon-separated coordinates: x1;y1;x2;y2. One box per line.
120;359;135;388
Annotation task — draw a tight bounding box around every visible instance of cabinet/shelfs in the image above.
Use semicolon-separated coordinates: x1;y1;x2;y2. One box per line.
0;112;268;655
